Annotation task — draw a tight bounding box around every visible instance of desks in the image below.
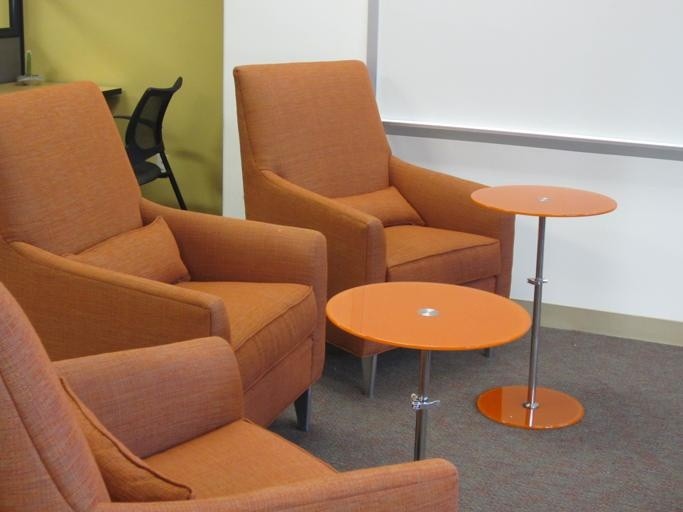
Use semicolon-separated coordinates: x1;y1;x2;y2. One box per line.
323;181;619;462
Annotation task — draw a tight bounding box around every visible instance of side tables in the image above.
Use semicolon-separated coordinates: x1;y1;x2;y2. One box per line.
471;184;617;430
325;280;534;460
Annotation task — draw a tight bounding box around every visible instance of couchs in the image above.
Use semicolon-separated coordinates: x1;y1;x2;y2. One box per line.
232;60;515;396
0;78;460;509
